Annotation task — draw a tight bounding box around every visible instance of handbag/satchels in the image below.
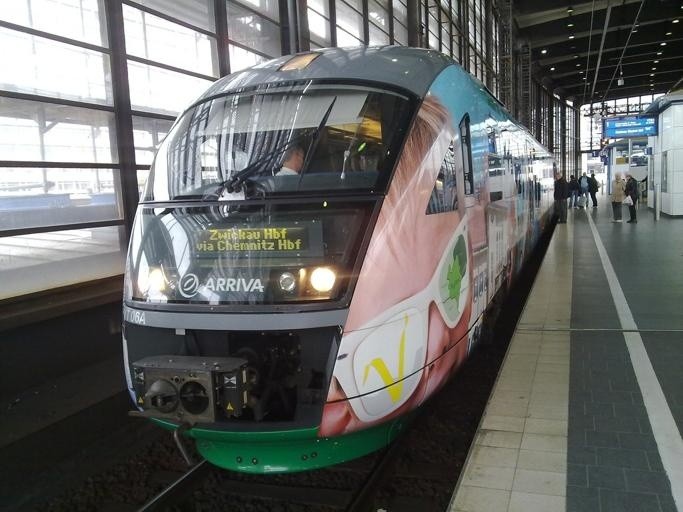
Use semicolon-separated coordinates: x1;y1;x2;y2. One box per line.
623;194;634;207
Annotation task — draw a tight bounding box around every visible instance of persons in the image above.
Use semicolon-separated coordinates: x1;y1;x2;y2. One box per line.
277;145;305;176
555;172;638;224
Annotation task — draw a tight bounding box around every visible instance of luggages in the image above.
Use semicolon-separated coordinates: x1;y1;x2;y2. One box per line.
578;188;587;207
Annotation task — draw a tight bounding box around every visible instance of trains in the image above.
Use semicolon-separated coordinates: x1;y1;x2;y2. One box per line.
121;44;566;476
